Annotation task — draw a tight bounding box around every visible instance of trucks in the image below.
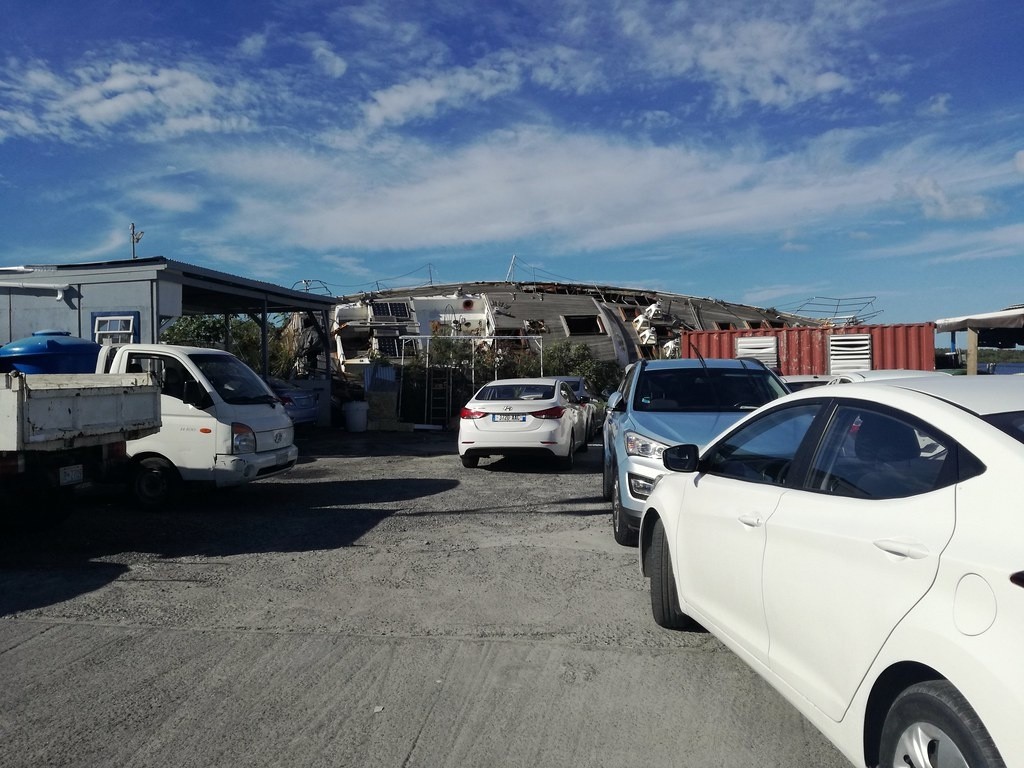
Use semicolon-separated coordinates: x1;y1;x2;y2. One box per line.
0;369;168;530
94;338;300;514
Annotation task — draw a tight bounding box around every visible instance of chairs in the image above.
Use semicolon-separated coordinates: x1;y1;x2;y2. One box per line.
541;390;555;399
851;418;933;501
642;376;679;412
499;388;516;399
716;374;751;409
163;367;189;398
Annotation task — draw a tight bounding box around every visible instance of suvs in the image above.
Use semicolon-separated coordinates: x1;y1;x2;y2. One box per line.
600;356;814;548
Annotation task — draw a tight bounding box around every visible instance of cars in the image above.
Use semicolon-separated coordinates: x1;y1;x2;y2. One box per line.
826;369;952;385
778;375;835;394
458;377;591;468
637;372;1023;766
228;373;319;423
518;375;607;443
